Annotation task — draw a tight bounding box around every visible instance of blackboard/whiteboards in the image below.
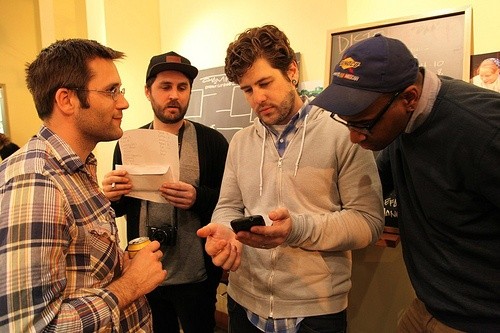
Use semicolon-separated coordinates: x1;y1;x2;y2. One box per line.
326;5;473;235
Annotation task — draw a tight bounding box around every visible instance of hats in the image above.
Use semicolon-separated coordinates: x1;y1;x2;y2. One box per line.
146;51;198;84
308;33;418;114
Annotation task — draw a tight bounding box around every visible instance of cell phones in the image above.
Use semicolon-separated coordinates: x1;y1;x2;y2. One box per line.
231;214;266;234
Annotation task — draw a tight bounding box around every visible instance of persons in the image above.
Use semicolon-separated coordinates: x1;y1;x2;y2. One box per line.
325;31;499;333
195;24;386;332
468;57;500;93
101;49;232;333
0;133;21;161
0;37;170;333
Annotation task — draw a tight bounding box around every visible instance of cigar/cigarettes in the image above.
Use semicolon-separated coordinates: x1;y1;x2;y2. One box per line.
111;182;115;190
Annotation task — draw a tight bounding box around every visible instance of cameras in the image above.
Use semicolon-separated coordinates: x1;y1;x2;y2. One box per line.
147;224;177;244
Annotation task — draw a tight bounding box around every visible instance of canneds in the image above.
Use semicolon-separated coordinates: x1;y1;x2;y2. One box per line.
126;236;153;260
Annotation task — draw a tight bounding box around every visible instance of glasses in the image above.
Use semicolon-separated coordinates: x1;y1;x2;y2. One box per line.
330;87;405;129
65;87;125;101
